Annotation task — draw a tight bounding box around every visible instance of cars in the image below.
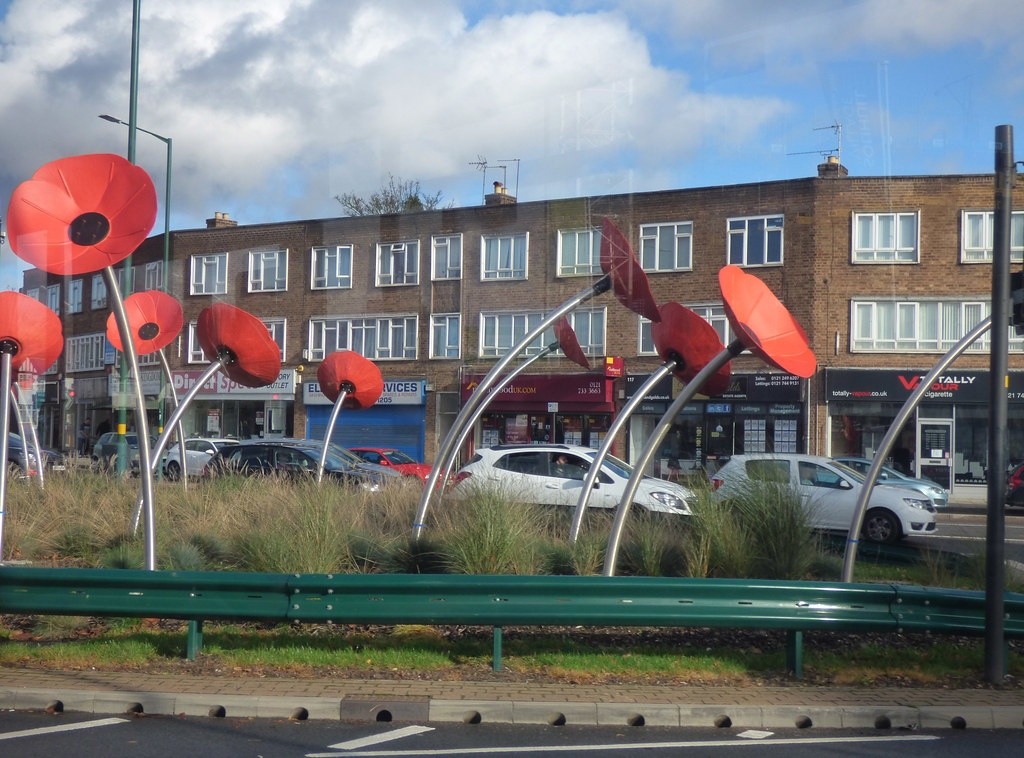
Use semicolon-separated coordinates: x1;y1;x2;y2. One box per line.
573;446;698;503
1003;462;1024;507
342;447;454;491
707;451;940;544
810;456;948;509
166;438;240;481
288;440;404;480
7;432;67;473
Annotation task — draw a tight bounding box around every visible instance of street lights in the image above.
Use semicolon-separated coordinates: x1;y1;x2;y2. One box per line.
101;115;172;480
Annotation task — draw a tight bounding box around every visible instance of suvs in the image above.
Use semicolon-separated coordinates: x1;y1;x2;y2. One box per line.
91;432;168;469
200;440;403;492
444;444;696;523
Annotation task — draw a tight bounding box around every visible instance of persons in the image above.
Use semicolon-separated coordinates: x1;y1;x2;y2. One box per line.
553;453;569;477
95;419;111;436
79;417;91;455
